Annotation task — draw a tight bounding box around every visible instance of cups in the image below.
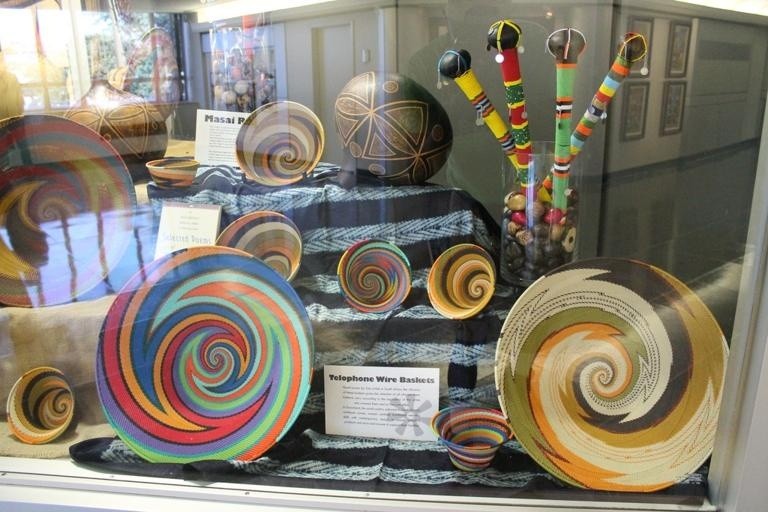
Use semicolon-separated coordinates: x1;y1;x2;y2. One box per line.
431;405;513;472
7;367;75;447
145;156;199;190
332;238;498;322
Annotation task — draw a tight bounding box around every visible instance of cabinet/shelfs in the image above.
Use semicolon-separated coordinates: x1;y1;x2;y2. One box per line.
4;161;717;510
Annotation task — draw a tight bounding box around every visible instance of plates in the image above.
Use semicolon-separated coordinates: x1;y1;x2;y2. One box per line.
0;115;137;307
494;257;731;493
93;244;315;467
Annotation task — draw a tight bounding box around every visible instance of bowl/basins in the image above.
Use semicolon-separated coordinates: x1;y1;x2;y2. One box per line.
215;102;326;284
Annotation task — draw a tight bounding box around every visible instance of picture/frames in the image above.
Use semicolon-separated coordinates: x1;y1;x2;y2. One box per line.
660;80;687;136
664;18;693;78
619;81;649;142
625;13;653;79
